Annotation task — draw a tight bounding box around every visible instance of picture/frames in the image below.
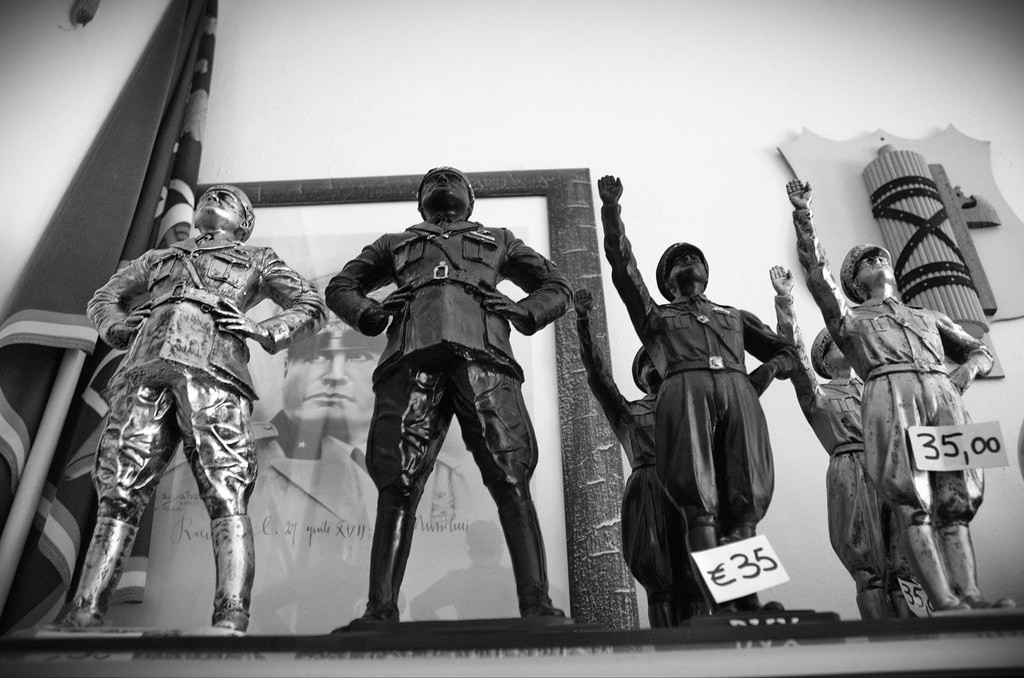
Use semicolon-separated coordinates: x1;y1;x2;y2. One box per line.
133;168;644;638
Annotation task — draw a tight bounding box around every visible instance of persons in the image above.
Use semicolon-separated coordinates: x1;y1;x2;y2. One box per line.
54;164;1015;635
